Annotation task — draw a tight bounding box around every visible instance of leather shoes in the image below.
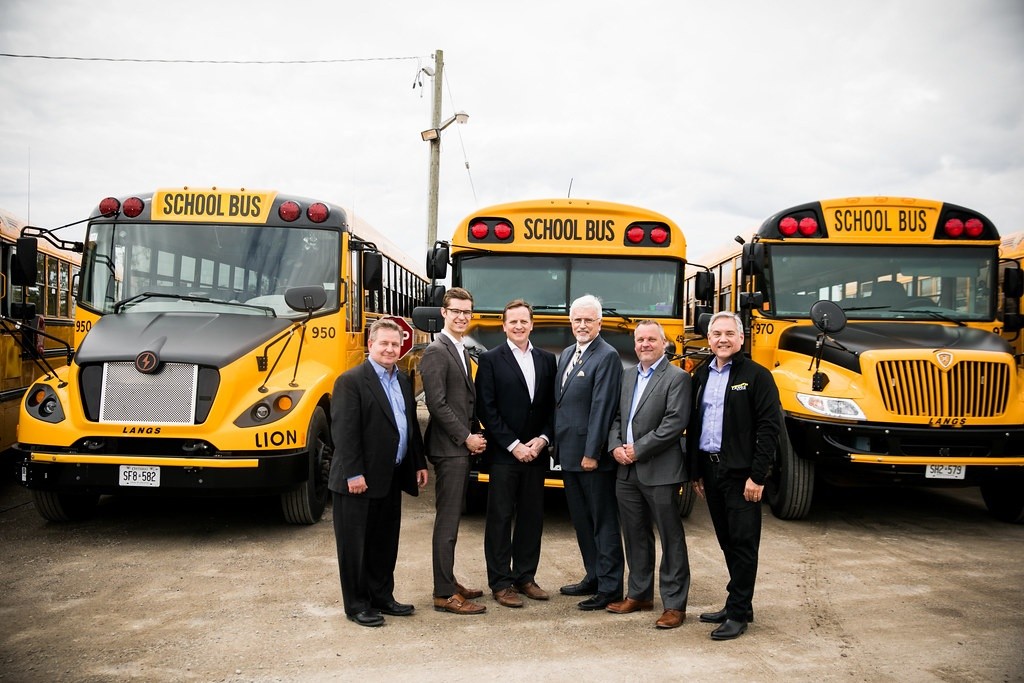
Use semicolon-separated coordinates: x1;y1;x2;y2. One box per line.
512;580;549;600
700;605;753;623
454;582;484;599
655;609;686;627
346;609;385;627
491;586;523;607
560;580;596;595
605;595;654;614
433;593;487;615
375;599;415;616
711;618;748;640
577;591;623;611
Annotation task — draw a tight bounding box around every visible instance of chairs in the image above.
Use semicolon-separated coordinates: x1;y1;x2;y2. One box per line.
872;281;906;308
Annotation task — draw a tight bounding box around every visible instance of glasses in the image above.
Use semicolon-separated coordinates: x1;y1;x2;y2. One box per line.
572;317;600;324
445;307;472;316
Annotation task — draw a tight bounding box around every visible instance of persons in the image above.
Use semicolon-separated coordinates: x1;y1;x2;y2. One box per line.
684;310;782;640
327;318;429;627
474;299;558;607
554;295;625;611
418;287;488;615
605;319;693;629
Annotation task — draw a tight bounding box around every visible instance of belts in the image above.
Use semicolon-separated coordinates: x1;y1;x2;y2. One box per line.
699;453;721;463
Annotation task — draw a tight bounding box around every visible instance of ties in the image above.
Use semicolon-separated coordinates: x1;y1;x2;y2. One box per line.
561;347;581;393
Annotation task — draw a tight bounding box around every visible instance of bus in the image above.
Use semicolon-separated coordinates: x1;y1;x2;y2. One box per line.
683;196;1024;522
0;207;90;454
412;197;716;515
10;186;447;526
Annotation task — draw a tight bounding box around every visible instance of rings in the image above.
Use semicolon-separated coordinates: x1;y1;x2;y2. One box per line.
754;496;758;498
622;461;624;464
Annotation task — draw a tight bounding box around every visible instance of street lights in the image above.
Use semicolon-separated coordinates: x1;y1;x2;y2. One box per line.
427;111;470;283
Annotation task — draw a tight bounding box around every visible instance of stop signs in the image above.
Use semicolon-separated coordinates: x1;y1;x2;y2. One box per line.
381;316;414;360
